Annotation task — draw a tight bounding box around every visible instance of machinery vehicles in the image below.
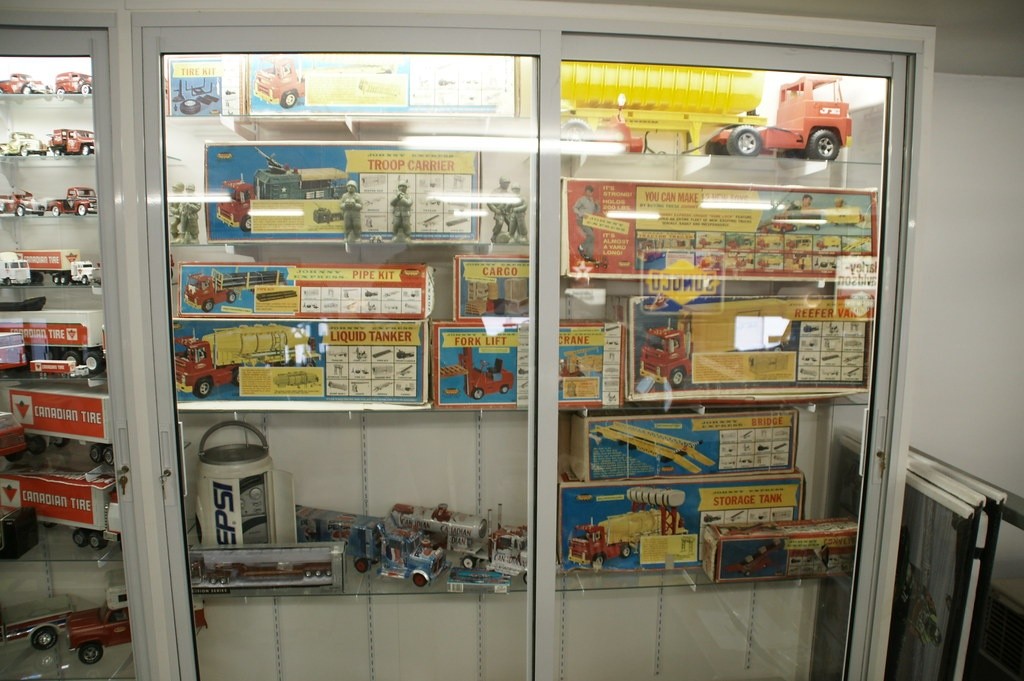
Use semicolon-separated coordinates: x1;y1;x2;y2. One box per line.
558;58;853;164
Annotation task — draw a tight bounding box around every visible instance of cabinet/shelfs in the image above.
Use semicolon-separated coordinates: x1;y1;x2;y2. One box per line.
534;2;937;681
124;0;537;681
0;0;152;681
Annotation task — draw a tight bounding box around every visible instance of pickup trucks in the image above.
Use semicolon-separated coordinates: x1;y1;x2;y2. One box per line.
45;188;98;216
0;596;75;652
63;585;207;665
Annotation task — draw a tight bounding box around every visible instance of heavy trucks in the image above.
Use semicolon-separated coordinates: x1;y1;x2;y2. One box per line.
184;265;287;313
170;321;323;397
195;558;331;588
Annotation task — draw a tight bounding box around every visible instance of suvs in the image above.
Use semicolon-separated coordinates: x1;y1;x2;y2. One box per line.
49;128;94;156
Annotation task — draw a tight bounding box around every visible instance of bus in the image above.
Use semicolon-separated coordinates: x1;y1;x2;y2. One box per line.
767;211;820;235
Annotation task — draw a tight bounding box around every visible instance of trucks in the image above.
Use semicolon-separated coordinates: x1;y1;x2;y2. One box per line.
0;412;27;464
14;250;98;286
0;330;27;371
295;502;450;590
564;486;689;573
637;300;795;387
385;503;533;592
0;251;32;285
214;147;350;233
9;383;115;466
252;55;393;109
0;464;126;553
0;319;109;379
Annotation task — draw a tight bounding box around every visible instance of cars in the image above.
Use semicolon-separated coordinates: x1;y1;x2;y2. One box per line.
0;74;44;94
0;132;51;156
1;185;45;219
56;72;92;94
462;357;512;400
